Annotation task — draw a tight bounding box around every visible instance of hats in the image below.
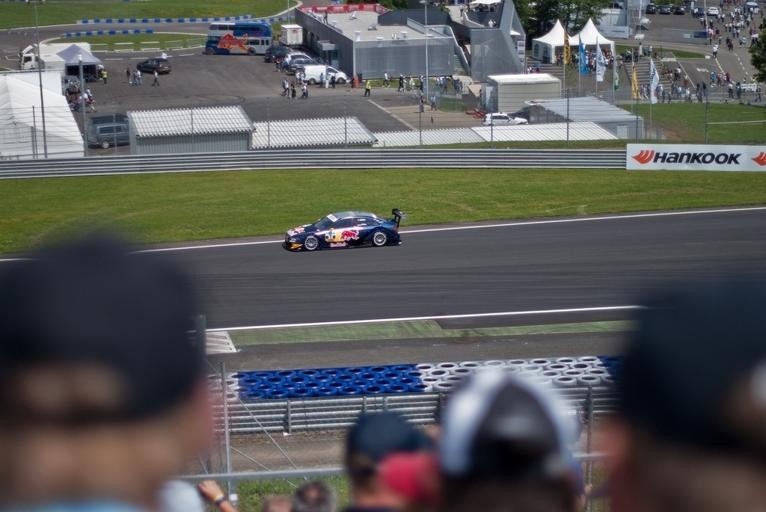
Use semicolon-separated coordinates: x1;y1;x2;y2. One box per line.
3;235;204;425
448;372;577;480
349;410;430;482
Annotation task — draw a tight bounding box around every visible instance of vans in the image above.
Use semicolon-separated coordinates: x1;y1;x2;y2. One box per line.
88;115;129;148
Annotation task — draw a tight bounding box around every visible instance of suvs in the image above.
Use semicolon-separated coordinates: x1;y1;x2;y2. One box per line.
482;113;528;125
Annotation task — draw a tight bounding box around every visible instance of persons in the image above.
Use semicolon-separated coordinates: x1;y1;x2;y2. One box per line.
604;277;765;512
0;227;213;511
152;70;161;88
345;366;587;511
96;64;142;87
320;73;336;89
282;78;308;100
75;89;92;107
383;71;463;112
363;78;371;97
526;65;540;73
162;51;167;59
559;0;766;105
159;480;334;512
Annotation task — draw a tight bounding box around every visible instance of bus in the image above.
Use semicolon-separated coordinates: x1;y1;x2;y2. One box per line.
205;22;273;56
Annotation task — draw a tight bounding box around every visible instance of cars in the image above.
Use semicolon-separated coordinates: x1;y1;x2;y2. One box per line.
707;6;718;16
646;4;684;14
264;44;346;86
136;58;172;74
281;208;402;253
